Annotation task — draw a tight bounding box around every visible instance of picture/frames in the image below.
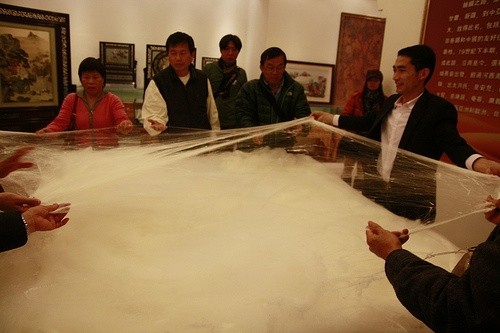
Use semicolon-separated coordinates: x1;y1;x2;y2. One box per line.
0;3;75;120
146;45;197;85
202;57;218;70
99;42;137;83
284;60;335;103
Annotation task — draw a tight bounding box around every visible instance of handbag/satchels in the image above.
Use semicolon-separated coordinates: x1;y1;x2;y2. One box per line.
63;93;78;145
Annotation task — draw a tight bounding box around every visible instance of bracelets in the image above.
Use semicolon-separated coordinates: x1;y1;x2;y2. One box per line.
22;216;30;236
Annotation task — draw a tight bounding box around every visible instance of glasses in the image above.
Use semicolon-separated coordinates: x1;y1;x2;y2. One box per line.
263;67;285;73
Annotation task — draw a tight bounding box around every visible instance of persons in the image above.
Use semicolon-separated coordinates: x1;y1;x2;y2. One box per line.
340;70;388;189
204;33;247;151
0;146;41;213
140;32;220;145
32;57;133;150
366;195;500;333
0;203;71;253
234;47;311;150
310;43;500;225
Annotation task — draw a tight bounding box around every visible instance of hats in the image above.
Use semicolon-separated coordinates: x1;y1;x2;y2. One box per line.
366;70;384;81
